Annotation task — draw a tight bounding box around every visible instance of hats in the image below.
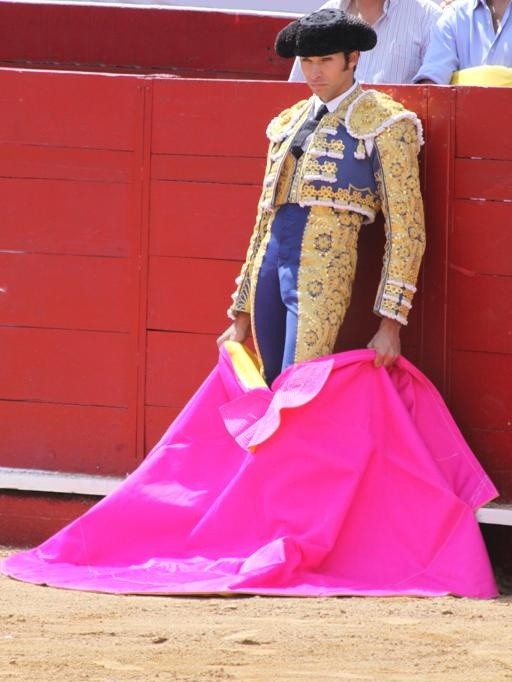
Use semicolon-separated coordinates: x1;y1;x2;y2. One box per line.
274;9;377;58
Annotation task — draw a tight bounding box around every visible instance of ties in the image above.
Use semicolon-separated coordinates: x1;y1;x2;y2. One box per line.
291;104;328;160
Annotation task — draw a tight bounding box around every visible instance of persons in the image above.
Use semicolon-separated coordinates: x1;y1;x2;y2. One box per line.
215;8;425;389
286;0;511;85
